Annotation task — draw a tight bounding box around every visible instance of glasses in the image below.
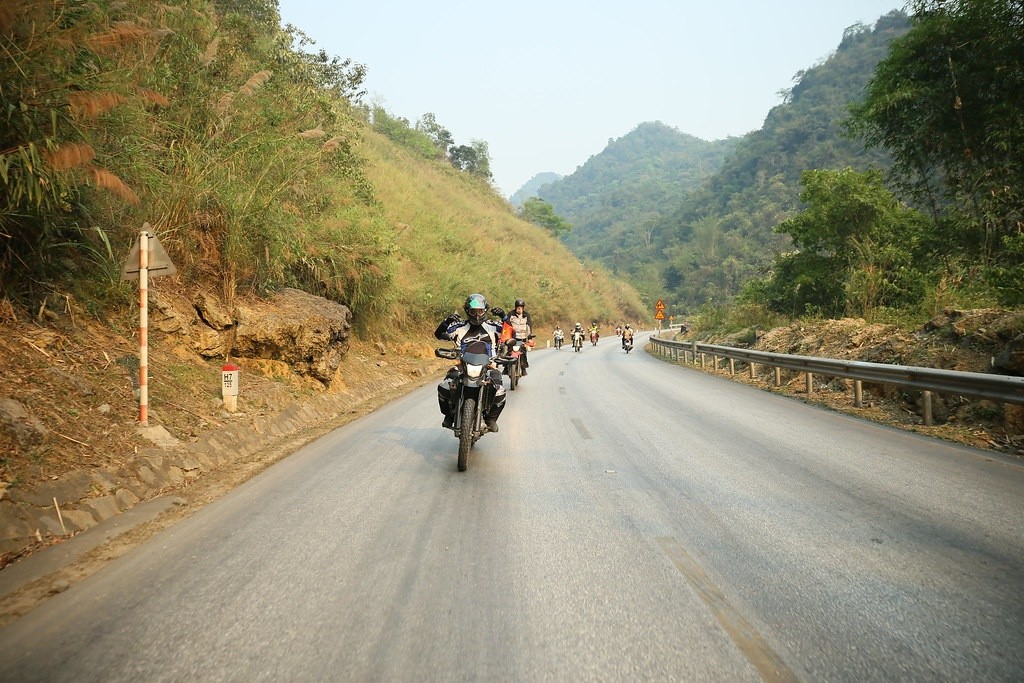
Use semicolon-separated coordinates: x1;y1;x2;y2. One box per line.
517;305;523;307
466;308;486;317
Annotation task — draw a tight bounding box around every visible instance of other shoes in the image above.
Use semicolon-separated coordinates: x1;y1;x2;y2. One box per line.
580;346;582;347
522;367;526;375
622;346;624;349
485;417;498;432
502;367;508;374
442;414;454;427
572;346;574;347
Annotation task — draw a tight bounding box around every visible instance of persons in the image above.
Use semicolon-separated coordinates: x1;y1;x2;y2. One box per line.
588;323;599;342
622;324;634;349
502;299;532;375
553;326;564;347
571;323;585;348
616;324;622;335
435;294;515;432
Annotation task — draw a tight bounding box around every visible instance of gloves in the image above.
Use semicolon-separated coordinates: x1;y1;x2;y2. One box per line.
492;308;506;318
446;314;461;325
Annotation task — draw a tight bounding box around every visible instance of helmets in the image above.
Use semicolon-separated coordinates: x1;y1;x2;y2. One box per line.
592;323;596;328
515;299;524;311
625;324;629;329
576;323;581;329
464;294;489;325
557;327;560;329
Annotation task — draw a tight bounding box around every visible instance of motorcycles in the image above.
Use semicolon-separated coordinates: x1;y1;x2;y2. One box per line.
621;333;634;354
616;327;621;337
492;331;536;391
436;331;523;473
571;330;585;352
587;328;600;346
552;332;563;350
